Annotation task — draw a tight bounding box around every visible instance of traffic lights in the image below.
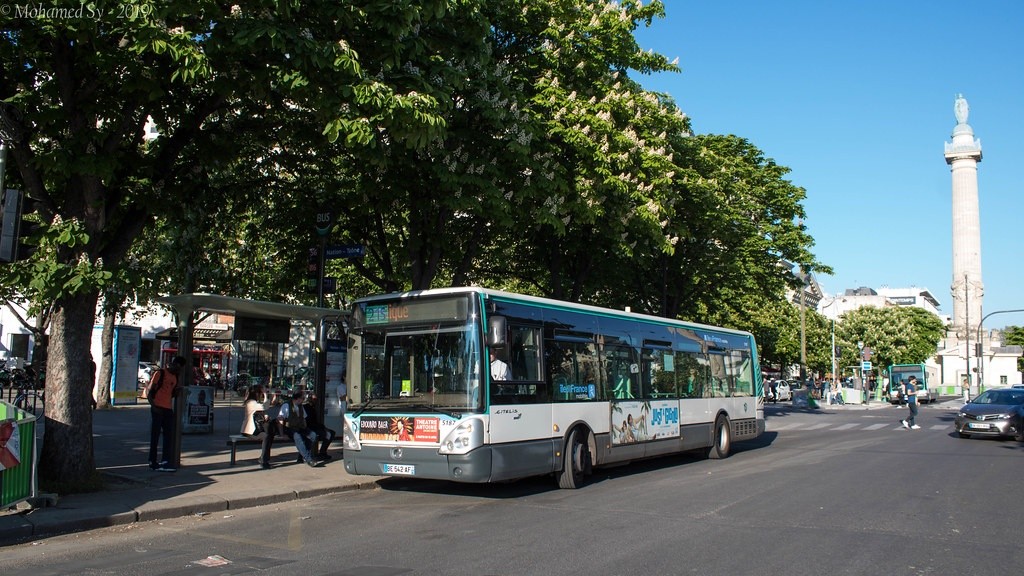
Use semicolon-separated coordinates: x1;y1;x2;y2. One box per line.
934;355;943;364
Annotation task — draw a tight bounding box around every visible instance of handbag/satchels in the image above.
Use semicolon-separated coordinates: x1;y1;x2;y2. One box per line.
252;412;265;434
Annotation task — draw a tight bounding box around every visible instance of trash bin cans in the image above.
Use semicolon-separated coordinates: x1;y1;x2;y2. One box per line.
792;388;809;407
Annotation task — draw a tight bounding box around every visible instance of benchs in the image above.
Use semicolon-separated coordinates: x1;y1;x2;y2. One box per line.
226;427;335;466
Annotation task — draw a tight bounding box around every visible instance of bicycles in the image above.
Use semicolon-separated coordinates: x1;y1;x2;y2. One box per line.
10;368;47;422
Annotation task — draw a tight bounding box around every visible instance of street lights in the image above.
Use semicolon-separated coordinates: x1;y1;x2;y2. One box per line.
951;272;986;401
822;297;847;315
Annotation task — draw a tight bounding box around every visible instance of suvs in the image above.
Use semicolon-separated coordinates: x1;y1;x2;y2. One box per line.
138;362;161;384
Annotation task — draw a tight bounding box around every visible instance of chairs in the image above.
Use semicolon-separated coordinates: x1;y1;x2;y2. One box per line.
551;369;753;398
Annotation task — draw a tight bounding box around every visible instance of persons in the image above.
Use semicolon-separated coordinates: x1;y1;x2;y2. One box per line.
769;378;778;404
902;375;920;430
240;385;273;469
89;350;98;410
303;393;336;460
276;390;324;468
913;380;920;407
822;377;842;403
897;380;909;409
614;414;656;444
961;378;972;405
336;368;347;423
684;363;712;396
763;378;770;405
146;355;185;472
189;390;210;425
473;347;512;395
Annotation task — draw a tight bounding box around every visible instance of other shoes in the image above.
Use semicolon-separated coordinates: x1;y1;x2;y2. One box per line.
911;424;921;429
318;453;333;459
897;405;903;409
906;403;909;407
903;420;909;429
308;459;325;468
149;461;176;471
257;457;272;469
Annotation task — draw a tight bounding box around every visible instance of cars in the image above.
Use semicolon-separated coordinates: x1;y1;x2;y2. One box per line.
954;389;1024;443
1011;384;1024;389
762;379;791;401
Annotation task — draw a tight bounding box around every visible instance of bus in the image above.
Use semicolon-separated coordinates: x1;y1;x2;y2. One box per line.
888;364;937;404
343;285;766;489
842;366;878;398
